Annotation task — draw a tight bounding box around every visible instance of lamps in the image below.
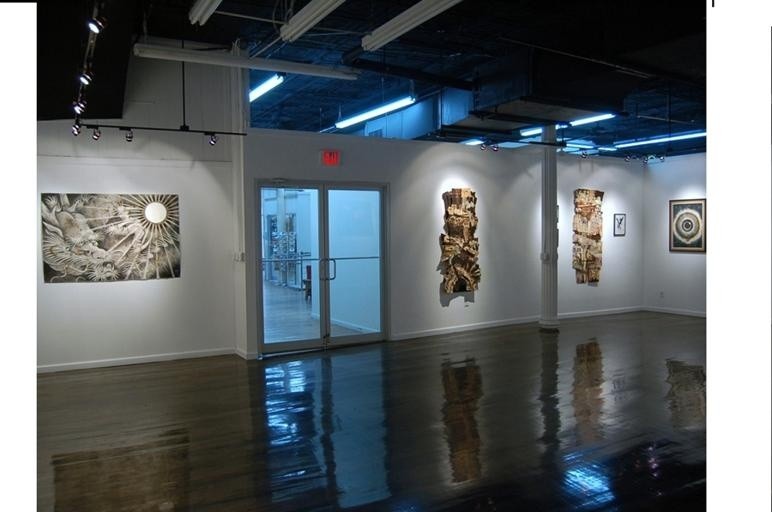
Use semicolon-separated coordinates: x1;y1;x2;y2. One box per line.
206;131;219;146
71;0;135;142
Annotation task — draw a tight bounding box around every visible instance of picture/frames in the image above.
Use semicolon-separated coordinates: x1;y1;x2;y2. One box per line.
669;199;706;252
614;214;626;236
